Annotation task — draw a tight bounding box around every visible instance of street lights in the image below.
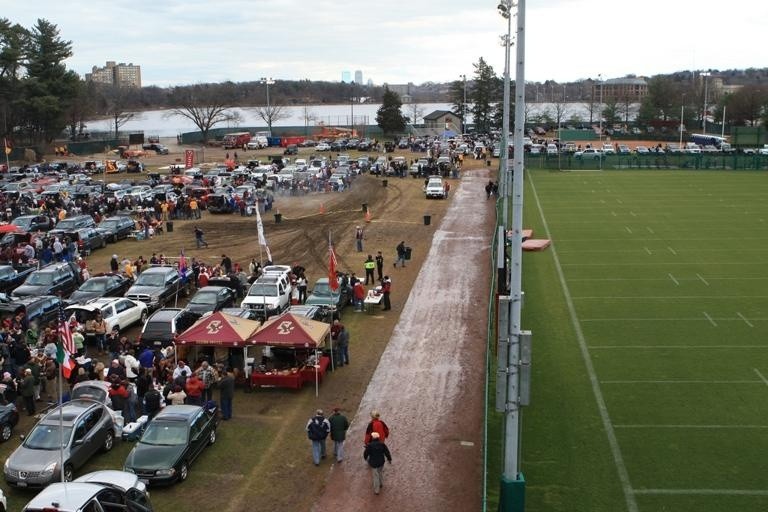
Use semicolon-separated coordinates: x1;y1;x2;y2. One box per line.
598;73;603;170
258;77;276;137
350;81;355;137
458;74;468;133
700;72;712;134
680;92;688;149
490;0;535;508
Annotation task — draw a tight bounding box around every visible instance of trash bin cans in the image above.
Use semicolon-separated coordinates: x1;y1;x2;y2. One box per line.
486;161;490;166
382;180;387;186
166;222;173;232
404;247;411;260
271;214;281;224
360;203;368;213
423;216;430;225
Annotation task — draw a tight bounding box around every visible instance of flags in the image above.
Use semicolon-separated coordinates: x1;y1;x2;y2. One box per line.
4;137;12;154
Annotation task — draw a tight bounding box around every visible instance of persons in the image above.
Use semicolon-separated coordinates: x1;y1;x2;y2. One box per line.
0;144;410;423
364;409;390;448
363;431;392;495
328;406;349;462
305;407;331;467
410;132;499;200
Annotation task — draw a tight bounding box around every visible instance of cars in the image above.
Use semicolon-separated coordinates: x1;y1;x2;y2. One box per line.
425;177;445;198
19;469;154;512
0;212;135;256
0;263;352;349
121;403;221;489
508;123;768;161
279;137;501;191
0;402;20;443
3;381;116;492
1;125;283;212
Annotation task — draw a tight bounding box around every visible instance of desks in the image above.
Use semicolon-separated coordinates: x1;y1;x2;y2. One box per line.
252;355;331;392
364;290;384;315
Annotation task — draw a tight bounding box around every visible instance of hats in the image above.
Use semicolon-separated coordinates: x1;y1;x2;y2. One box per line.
371;432;380;438
371;412;380;418
